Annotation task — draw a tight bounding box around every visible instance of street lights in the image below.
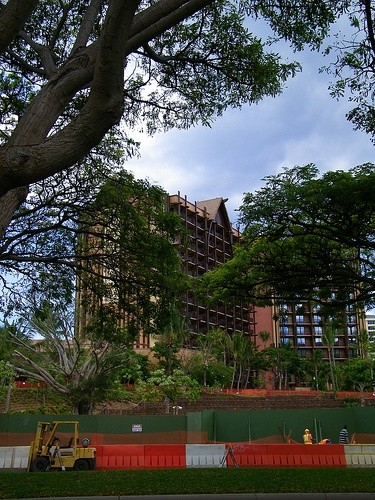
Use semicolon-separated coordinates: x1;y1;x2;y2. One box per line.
172;405;182;416
349;338;356;358
328;338;338;391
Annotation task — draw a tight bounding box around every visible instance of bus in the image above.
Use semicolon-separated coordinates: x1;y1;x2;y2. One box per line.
12;375;44;388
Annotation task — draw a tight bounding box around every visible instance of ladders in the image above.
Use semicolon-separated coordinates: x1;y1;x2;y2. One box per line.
218;449;239;469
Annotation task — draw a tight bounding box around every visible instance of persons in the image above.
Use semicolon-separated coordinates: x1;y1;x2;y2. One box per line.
317;438;332;445
302;428;312;445
339;425;349;444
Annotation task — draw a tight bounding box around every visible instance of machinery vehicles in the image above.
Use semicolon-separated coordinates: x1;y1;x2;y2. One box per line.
25;420;97;471
